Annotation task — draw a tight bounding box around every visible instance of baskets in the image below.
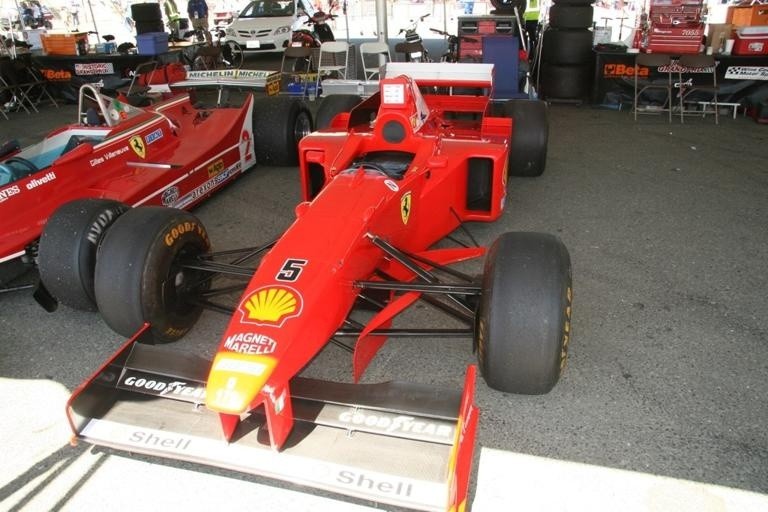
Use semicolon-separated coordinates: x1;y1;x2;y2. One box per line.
40;31;89;56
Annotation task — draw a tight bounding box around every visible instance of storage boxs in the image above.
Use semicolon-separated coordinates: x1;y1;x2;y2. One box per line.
732;25;768;54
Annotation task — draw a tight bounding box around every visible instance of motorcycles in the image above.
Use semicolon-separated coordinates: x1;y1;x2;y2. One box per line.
394;12;433;95
290;0;339;49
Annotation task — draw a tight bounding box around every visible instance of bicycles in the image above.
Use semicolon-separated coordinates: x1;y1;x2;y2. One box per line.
430;25;479;96
183;28;244;71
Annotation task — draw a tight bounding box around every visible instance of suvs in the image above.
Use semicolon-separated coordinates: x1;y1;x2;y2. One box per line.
0;5;54;34
213;6;240;25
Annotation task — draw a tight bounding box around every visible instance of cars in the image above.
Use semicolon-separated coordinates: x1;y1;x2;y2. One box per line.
218;0;323;63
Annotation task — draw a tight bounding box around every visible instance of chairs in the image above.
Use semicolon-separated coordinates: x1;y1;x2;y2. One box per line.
274;41;392;106
0;59;59;120
114;61;158;107
628;54;720;125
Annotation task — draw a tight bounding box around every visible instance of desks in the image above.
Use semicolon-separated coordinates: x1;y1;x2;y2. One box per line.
589;47;767;111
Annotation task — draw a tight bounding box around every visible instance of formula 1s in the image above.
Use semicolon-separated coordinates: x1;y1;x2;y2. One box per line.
0;60;574;512
0;57;318;347
